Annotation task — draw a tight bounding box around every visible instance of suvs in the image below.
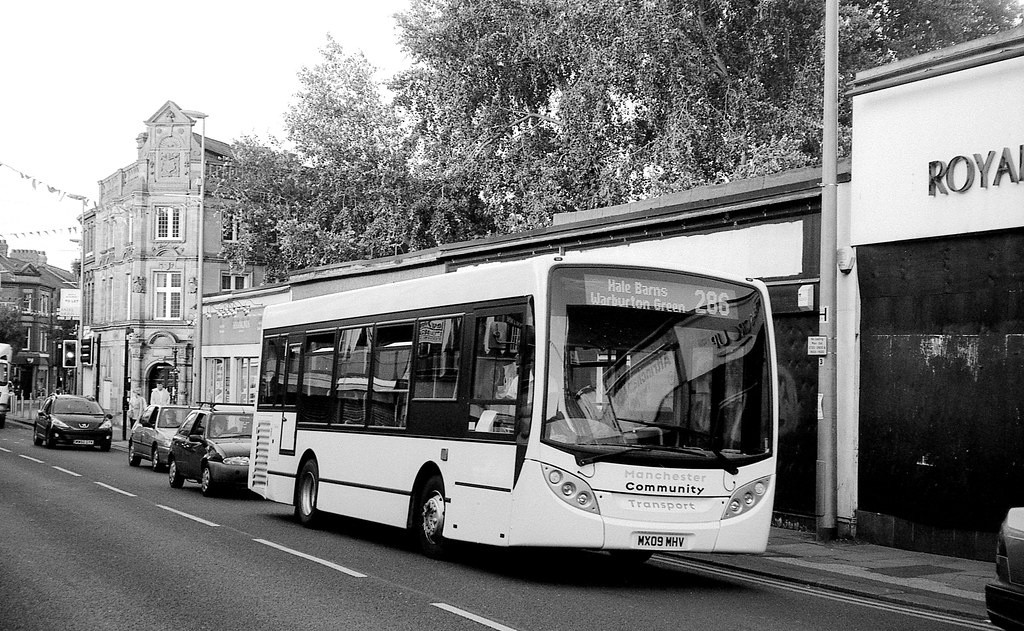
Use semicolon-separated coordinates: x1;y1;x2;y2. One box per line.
33;393;112;453
168;401;255;497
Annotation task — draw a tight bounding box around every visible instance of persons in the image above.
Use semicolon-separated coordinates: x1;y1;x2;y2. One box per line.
150;382;171;406
127;387;147;429
507;349;559;440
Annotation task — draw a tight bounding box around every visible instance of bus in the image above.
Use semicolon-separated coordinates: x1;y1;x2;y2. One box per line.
247;259;780;567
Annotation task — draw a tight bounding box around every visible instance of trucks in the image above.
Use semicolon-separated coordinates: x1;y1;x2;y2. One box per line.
0;343;13;428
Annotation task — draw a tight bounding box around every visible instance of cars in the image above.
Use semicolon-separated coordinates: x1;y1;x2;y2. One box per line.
127;403;206;473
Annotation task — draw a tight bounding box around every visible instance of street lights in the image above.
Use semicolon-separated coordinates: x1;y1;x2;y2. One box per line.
180;109;209;406
68;194;87;394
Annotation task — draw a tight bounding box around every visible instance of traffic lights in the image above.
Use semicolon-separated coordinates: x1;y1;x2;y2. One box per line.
62;340;77;369
80;335;93;366
